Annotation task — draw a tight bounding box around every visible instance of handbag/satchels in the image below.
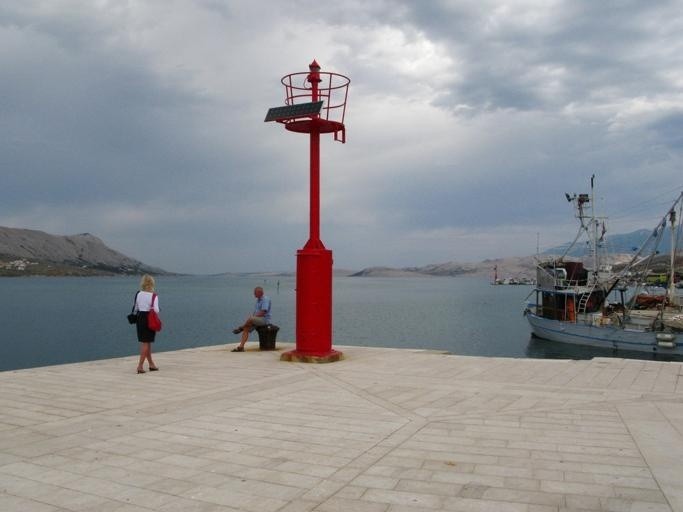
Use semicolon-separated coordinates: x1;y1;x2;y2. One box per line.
148;309;161;331
128;314;140;324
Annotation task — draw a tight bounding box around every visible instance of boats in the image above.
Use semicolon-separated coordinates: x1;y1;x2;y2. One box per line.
495;274;537;286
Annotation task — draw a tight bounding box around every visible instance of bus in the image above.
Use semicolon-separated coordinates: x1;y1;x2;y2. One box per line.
667;273;680;282
645;274;666;283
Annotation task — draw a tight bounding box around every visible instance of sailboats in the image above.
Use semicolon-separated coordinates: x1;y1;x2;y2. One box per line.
522;174;683;356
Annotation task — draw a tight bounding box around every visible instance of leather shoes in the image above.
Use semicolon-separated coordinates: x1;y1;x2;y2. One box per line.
137;368;158;373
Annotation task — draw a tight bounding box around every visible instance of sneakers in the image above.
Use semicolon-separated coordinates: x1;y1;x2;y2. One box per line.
234;327;243;333
232;347;243;352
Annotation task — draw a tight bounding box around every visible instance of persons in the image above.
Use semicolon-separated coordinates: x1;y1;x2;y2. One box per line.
231;286;271;352
134;273;161;373
